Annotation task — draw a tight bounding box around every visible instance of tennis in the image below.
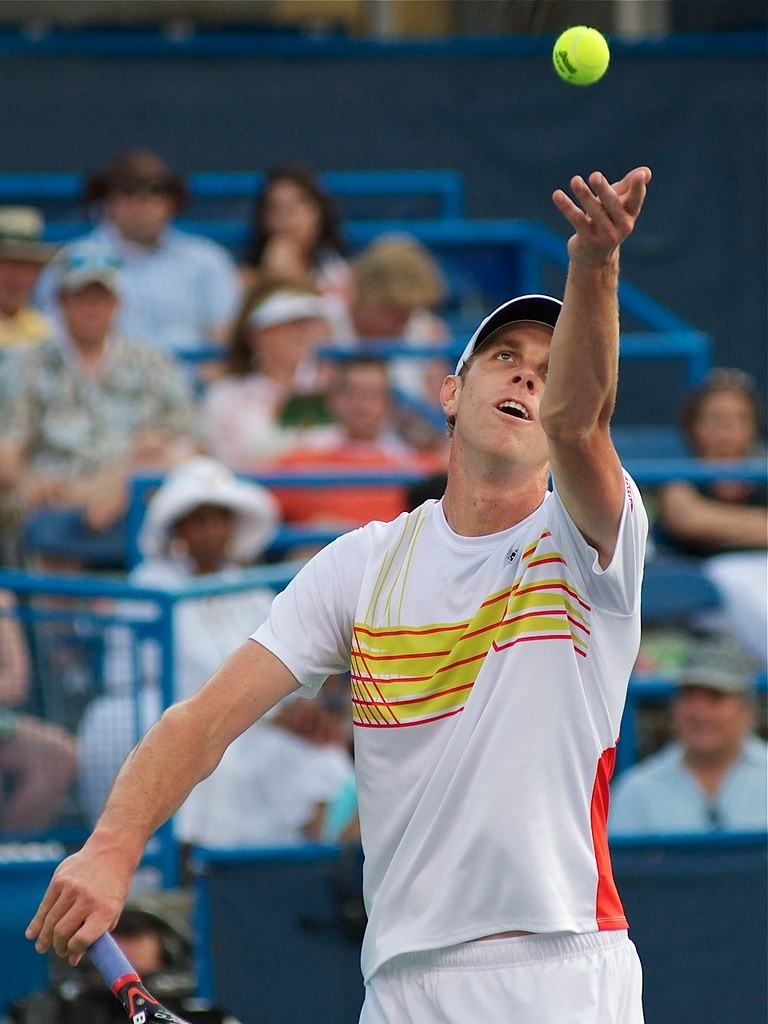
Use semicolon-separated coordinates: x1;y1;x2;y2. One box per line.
551;24;612;89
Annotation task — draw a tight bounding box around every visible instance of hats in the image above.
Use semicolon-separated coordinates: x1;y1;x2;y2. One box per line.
667;632;761;694
454;294;563;379
138;457;276;562
61;267;115;295
2;208;61;266
248;289;341;330
355;232;446;309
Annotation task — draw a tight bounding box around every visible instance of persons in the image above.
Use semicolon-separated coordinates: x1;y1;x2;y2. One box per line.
601;650;768;844
655;371;768;668
0;149;458;849
23;165;655;1024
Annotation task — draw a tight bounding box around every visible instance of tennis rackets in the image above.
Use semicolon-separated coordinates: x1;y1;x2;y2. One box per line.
86;930;204;1024
